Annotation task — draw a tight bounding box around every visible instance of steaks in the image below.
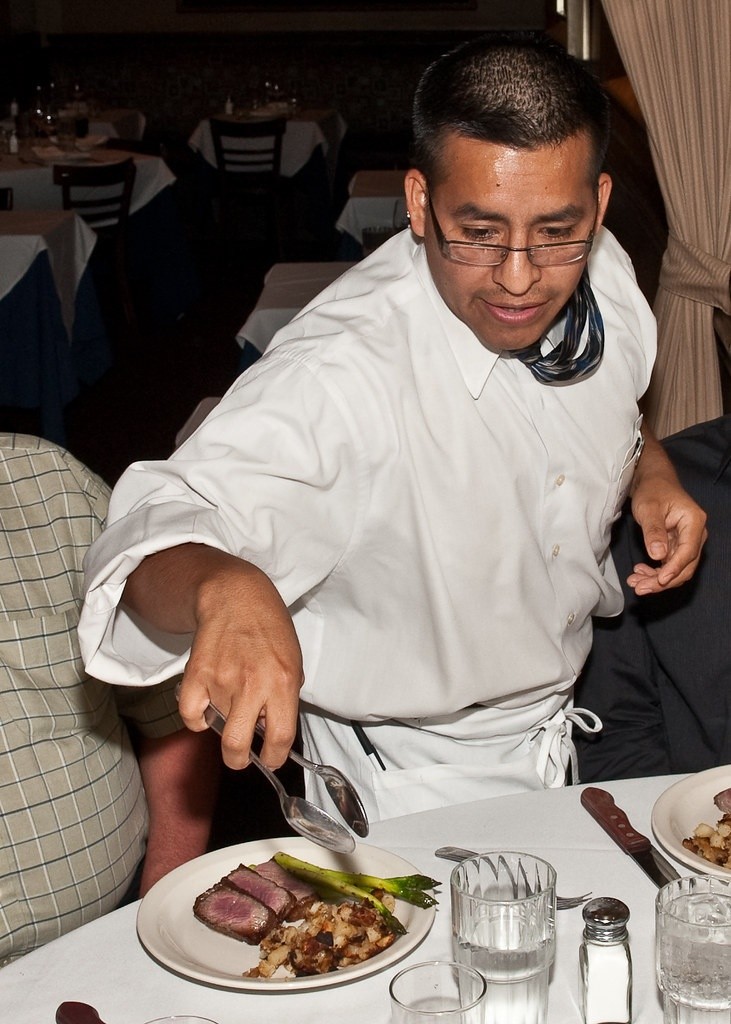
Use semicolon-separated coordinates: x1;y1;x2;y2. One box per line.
251;858;320;924
223;863;296;922
192;877;281;945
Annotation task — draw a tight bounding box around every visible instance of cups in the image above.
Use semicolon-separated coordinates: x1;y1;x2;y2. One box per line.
389;960;488;1024
655;874;731;1024
145;1014;219;1024
362;225;394;259
391;199;409;231
450;851;558;1024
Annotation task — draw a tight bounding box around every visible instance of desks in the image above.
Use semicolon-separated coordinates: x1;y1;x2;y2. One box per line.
333;169;415;246
238;263;354;370
0;211;111;417
0;139;181;232
3;107;149;152
174;396;220;449
185;104;353;206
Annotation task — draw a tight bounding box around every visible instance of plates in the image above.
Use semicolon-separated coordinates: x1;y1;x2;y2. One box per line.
651;764;731;876
135;835;435;991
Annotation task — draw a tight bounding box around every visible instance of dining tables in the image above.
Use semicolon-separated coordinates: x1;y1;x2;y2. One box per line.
0;770;702;1024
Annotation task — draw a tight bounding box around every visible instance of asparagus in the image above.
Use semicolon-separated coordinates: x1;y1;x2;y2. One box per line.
248;853;441;935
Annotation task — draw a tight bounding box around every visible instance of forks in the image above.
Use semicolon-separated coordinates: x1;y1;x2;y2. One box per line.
434;846;593;908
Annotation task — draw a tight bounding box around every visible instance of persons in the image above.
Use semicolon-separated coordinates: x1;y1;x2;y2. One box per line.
79;30;710;831
0;430;222;966
573;411;731;784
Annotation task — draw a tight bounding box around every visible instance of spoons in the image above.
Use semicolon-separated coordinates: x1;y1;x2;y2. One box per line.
255;720;369;839
174;679;356;854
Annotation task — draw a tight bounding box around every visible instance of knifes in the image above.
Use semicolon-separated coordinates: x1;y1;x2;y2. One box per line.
580;787;682;896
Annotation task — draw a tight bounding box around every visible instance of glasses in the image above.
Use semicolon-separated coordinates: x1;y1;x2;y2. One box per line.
427;184;599;266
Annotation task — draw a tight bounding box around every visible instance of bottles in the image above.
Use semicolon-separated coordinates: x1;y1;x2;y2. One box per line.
579;897;633;1024
224;99;235;115
0;129;8;155
7;129;19;154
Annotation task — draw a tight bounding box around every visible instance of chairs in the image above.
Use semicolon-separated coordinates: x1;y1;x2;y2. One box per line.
0;117;291;373
